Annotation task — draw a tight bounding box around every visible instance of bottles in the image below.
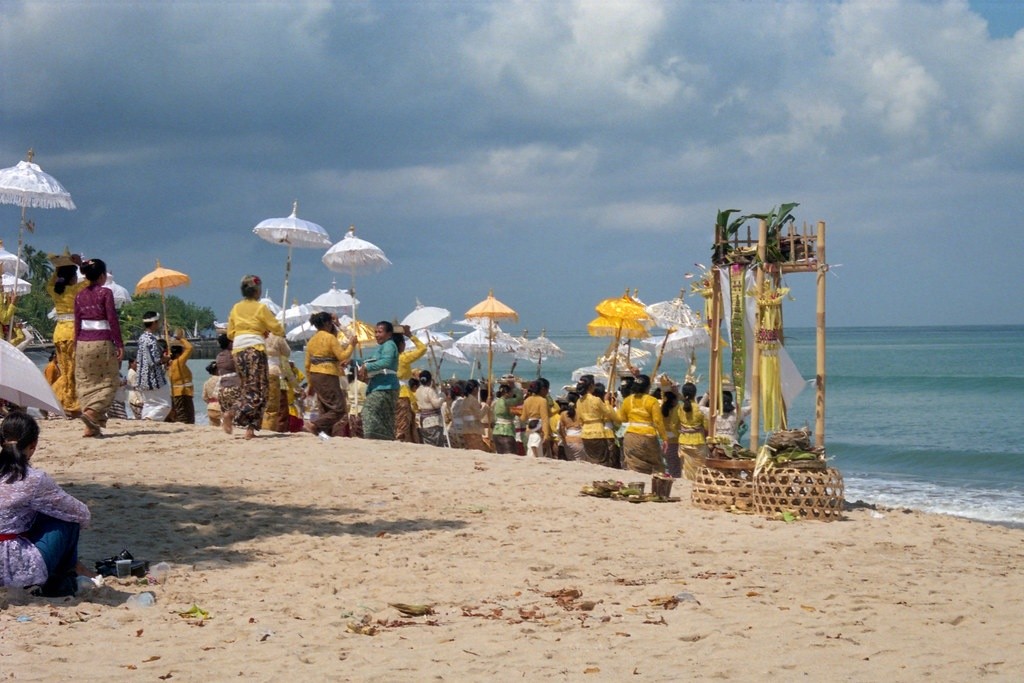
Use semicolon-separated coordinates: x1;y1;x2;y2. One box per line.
127;589;157;611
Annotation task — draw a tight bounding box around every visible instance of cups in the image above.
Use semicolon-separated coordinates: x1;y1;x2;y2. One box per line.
114;559;132;579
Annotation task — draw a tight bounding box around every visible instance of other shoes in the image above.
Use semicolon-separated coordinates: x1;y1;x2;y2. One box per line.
85;428;103;436
80;412;100;431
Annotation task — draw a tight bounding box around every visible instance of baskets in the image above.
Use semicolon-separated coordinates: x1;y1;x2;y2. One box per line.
691;466;754;515
752;467;843;523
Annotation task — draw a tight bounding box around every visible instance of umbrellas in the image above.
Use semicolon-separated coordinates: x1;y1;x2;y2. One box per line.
253;201;328;329
0;245;32;296
259;284;710;403
0;151;76;340
0;339;67;419
322;225;386;415
75;263;189;356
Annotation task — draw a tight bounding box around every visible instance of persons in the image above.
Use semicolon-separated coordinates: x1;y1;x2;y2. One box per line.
0;277;34;352
204;312;752;481
222;275;285;439
0;412;92;597
45;249;127;438
128;309;196;424
